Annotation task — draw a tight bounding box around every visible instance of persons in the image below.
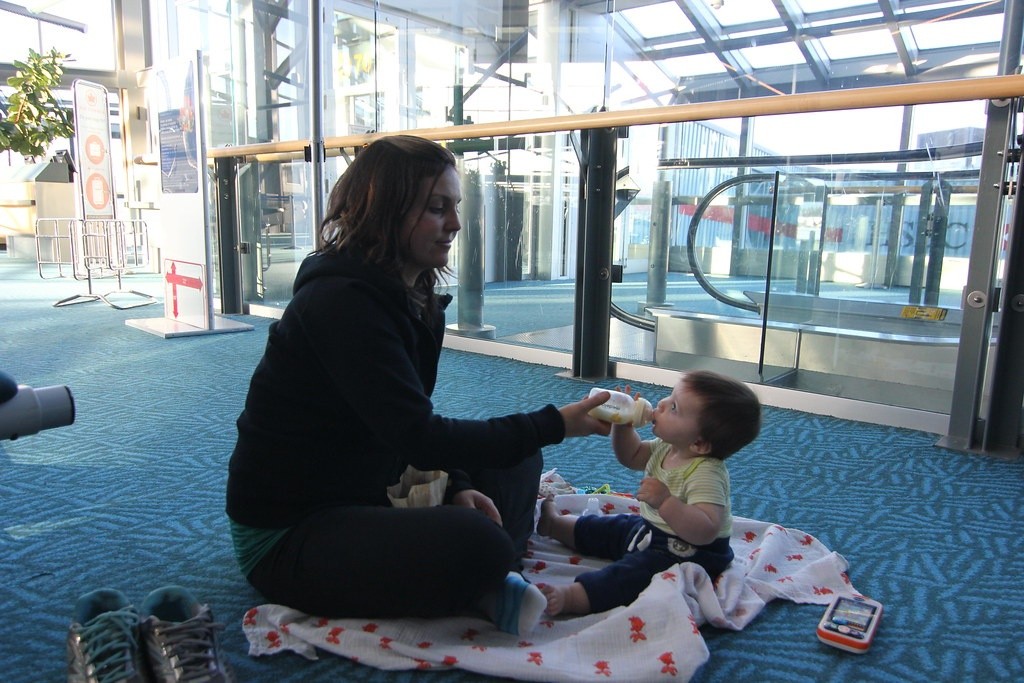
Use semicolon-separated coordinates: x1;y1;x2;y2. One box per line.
226;134;610;639
535;370;761;619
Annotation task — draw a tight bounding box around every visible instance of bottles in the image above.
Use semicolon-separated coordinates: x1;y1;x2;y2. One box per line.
587;387;653;428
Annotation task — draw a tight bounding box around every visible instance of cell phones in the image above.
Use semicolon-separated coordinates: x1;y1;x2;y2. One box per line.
816;593;883;653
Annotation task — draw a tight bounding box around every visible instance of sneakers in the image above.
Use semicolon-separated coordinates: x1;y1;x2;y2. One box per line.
137;584;235;683
66;587;144;683
